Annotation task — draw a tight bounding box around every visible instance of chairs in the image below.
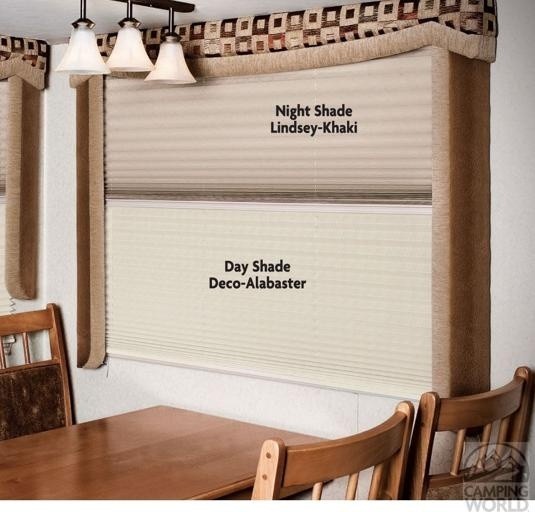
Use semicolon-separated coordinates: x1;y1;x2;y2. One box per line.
1;305;73;440
402;365;529;499
240;401;414;500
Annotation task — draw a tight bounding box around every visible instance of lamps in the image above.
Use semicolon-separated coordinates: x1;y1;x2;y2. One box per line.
51;0;207;90
